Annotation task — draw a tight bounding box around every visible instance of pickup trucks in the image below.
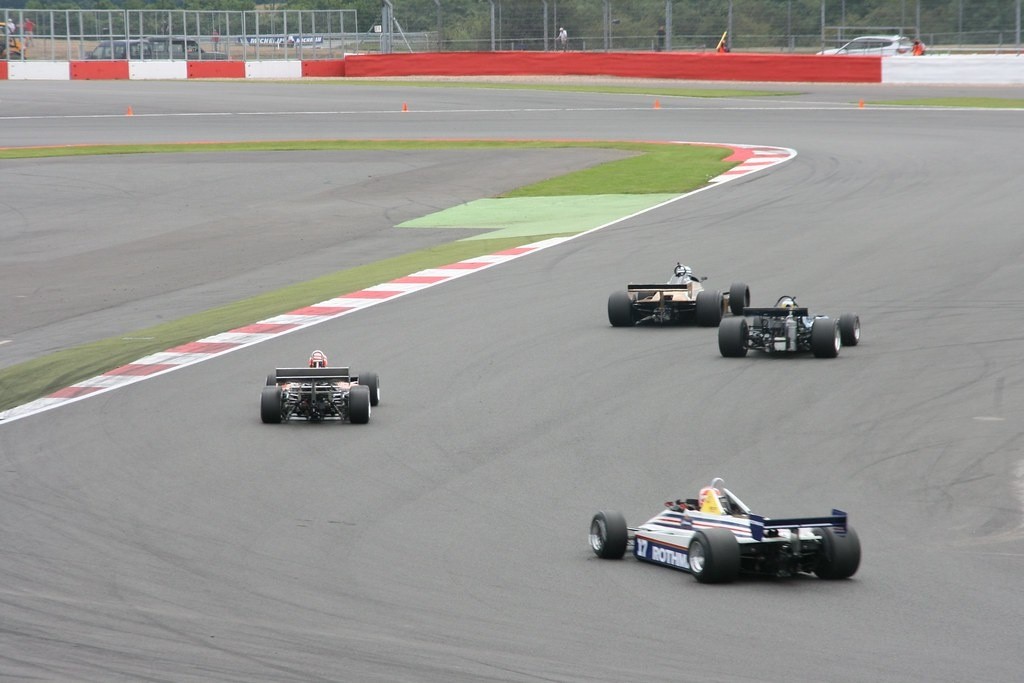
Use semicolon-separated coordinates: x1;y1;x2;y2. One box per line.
147;36;228;60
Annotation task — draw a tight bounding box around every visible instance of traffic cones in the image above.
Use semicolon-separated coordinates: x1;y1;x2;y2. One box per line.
654;100;661;109
127;106;135;115
858;99;865;109
402;101;409;112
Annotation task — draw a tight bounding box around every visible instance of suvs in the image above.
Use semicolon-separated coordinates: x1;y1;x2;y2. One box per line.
815;35;915;57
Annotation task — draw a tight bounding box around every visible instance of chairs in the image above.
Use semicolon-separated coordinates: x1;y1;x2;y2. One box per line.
310;350;324;367
773;296;799;307
709;477;731;514
670;262;687;284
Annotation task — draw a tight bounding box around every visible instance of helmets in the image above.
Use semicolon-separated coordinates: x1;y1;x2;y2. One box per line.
309;349;328;368
677;264;691;278
781;299;793;309
698;486;726;510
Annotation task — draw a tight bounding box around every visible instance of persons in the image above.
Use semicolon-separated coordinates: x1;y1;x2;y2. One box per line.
556;27;568;51
310;348;328;368
24;17;36;47
909;37;926;55
212;29;220;50
678;264;699;282
698;486;720;512
656;25;666;47
718;40;729;53
782;298;794;308
8;18;17;42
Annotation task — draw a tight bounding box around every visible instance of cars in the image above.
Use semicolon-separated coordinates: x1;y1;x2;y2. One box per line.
0;22;29;60
83;39;153;60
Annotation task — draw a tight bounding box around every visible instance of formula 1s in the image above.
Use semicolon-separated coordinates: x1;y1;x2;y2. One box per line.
717;294;862;359
588;477;862;585
607;262;751;326
260;350;380;425
